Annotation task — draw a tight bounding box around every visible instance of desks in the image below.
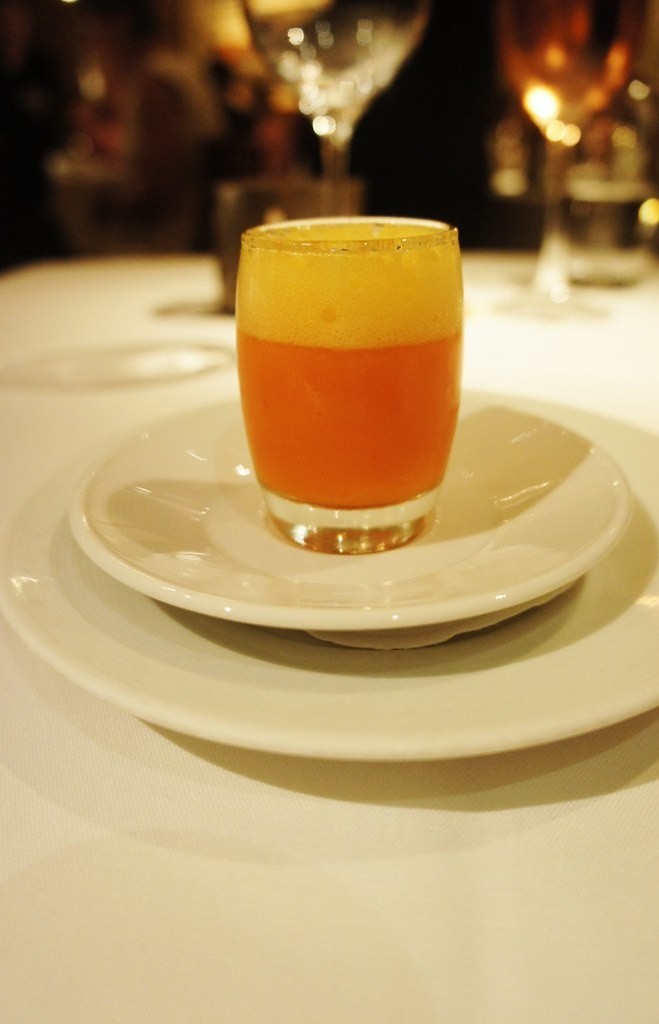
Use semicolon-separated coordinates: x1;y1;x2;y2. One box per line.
0;252;659;1024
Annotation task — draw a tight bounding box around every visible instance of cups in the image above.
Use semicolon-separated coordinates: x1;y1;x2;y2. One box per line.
235;216;463;555
215;179;364;315
554;119;638;286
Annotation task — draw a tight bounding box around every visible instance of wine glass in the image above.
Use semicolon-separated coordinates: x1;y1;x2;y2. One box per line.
497;0;639;307
244;0;428;216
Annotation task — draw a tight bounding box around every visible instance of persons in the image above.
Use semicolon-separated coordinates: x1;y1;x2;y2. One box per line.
199;0;543;254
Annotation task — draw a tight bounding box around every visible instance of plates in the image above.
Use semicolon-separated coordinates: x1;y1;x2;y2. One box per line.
9;388;659;760
66;388;630;651
